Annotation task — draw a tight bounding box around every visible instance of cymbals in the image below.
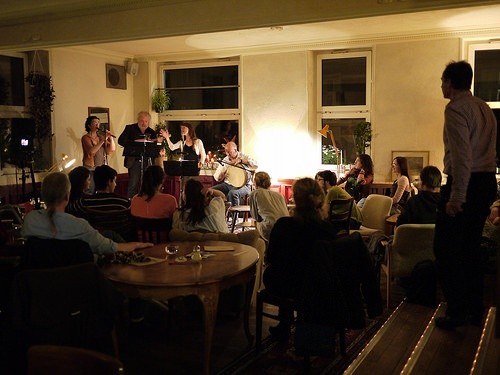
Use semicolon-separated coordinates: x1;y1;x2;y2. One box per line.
135;139;154;143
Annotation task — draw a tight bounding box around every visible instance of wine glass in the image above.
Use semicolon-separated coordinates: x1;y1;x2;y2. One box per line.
203;151;222;170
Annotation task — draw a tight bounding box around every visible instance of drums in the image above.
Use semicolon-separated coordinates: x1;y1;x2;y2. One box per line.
225;164;248;188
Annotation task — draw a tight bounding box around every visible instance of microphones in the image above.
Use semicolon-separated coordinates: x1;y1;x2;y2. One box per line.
181;131;187;144
218;161;224;166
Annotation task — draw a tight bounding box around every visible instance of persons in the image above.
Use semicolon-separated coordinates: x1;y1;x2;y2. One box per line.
211;141;258;227
248;172;289;244
434;61;500;329
117;111;163;198
264;178;337;339
159;122;206;175
395;165;447;228
336;154;374;204
65;165;132;213
389;156;410;211
68;166;91;200
130;164;177;245
82;115;115;171
316;170;363;226
171;179;230;234
21;172;154;257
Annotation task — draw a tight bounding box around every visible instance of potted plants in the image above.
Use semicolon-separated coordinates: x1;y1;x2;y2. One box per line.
151;90;170;113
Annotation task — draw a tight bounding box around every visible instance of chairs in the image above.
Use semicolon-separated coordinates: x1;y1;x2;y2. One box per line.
0;194;438;375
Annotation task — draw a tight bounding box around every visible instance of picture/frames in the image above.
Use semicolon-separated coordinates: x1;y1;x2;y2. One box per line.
392;150;429;183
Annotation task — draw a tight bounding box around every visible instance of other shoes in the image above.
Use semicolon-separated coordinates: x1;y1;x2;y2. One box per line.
436;308;482;332
130;300;160;322
268;323;292;337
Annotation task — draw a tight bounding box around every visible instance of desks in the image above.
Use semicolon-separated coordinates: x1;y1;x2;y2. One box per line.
229;205;251;233
98;241;260;375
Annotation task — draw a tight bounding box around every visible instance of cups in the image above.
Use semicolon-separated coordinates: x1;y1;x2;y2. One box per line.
166;244;179;254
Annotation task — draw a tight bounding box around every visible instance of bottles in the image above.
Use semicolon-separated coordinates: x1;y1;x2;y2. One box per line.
191;245;203;263
39;201;47;209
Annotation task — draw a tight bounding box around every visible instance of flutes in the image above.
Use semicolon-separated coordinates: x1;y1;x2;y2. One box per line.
96;127;116;138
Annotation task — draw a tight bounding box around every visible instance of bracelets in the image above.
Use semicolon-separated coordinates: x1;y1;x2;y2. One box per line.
239;158;242;163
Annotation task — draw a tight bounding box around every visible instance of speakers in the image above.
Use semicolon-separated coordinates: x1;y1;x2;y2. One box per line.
88;107;110;147
128;61;138;76
11;117;34;151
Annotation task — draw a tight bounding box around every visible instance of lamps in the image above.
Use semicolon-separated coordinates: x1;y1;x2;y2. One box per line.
315;124;340;180
